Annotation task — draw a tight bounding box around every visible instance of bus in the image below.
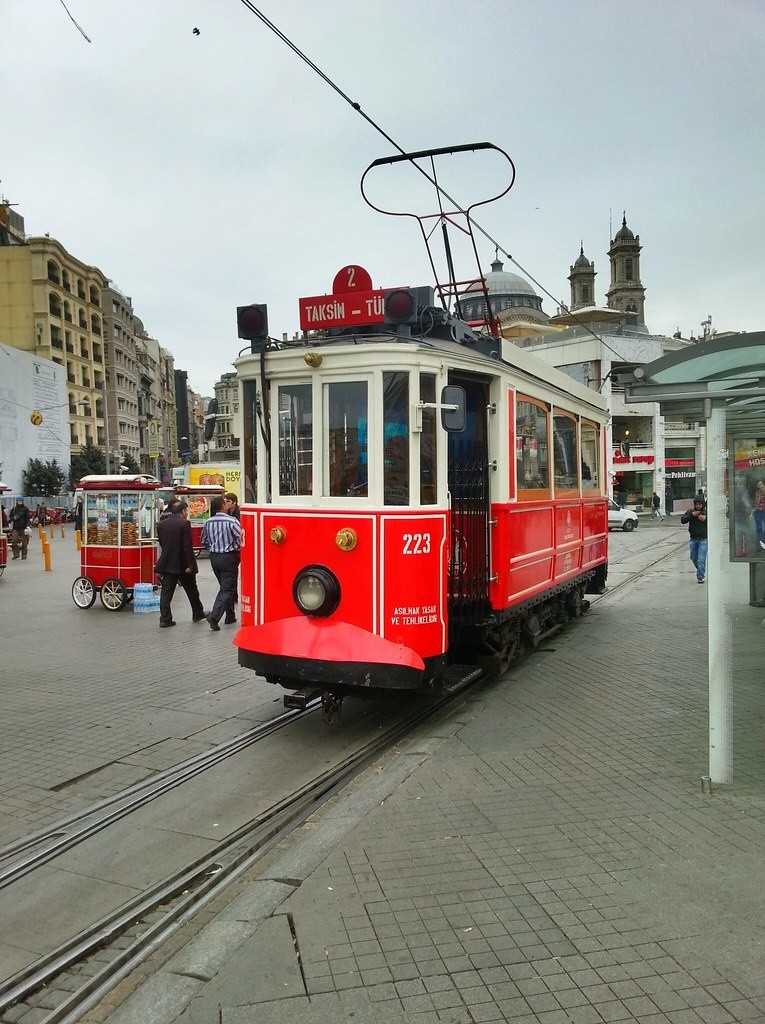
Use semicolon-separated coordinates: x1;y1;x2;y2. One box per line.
204;142;612;727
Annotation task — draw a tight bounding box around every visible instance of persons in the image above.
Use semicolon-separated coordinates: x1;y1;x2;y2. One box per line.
623;437;628;445
749;479;765;554
680;489;707;584
155;492;242;631
616;489;628;509
354;435;428;506
634;437;643;445
9;495;30;560
74;496;82;541
650;492;664;521
0;504;8;528
613;439;620;444
35;501;47;528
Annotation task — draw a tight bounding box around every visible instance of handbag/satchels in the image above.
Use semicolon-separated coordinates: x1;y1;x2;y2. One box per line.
9;521;13;530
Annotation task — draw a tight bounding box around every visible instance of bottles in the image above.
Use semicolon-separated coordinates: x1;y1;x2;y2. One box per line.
133;582;161;613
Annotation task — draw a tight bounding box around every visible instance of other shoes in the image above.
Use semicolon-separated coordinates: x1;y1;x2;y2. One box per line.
698;579;704;583
194;612;210;622
207;615;220;630
22;557;26;560
226;619;237;623
11;556;20;560
659;518;664;521
160;621;176;627
649;519;654;521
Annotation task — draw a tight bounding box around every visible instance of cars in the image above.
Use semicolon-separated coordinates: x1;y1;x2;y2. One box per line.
607;497;639;532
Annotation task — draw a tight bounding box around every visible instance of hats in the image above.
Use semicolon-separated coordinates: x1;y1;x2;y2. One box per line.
16;496;24;501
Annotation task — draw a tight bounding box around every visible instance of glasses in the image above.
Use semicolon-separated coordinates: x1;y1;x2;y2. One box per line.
226;501;231;503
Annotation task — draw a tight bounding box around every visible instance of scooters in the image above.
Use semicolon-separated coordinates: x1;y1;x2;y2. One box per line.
2;524;30;551
